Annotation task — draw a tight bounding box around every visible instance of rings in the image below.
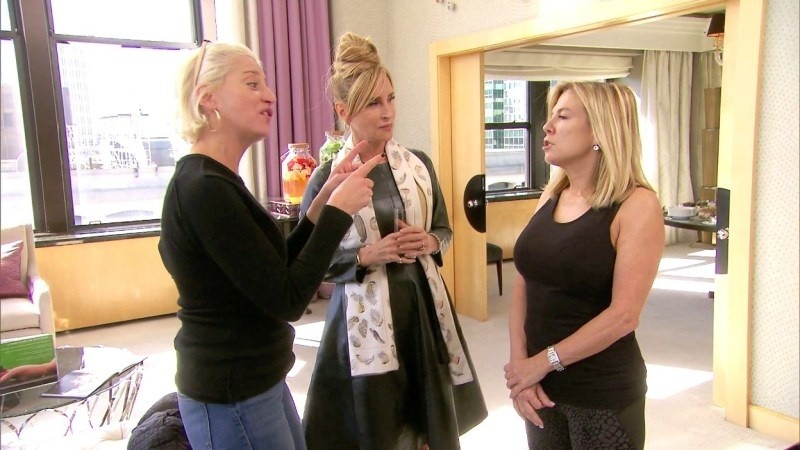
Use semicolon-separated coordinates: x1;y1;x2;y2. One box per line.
419;241;426;252
397;256;403;264
369;197;372;199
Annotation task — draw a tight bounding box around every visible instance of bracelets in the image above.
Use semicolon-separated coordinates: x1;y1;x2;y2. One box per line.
427;231;442;256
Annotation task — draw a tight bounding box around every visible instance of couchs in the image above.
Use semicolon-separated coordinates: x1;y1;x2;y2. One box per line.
0;223;59;350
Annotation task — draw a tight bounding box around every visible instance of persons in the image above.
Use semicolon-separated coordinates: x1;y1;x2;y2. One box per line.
158;41;387;450
503;81;664;450
298;32;488;450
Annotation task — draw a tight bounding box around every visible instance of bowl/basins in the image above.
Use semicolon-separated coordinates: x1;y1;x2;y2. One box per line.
318;282;335;297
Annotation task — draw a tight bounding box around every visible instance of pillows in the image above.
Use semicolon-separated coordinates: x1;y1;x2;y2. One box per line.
1;241;27;298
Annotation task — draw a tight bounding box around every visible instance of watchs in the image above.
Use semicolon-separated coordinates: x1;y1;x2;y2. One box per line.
546;344;566;372
354;253;364;270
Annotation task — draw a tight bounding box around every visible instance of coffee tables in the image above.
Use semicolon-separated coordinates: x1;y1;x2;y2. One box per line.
1;348;143;440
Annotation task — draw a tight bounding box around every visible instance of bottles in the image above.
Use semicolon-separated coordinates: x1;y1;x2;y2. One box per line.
282;144;318;204
319;131;346;165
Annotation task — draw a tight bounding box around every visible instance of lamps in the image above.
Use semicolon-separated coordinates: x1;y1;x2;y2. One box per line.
708;12;725;66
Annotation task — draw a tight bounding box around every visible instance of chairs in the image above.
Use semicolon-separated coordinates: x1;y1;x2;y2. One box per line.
486;243;503;298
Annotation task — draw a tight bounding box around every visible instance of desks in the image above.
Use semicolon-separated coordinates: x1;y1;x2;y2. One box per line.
271;196;328;304
665;213;719;236
